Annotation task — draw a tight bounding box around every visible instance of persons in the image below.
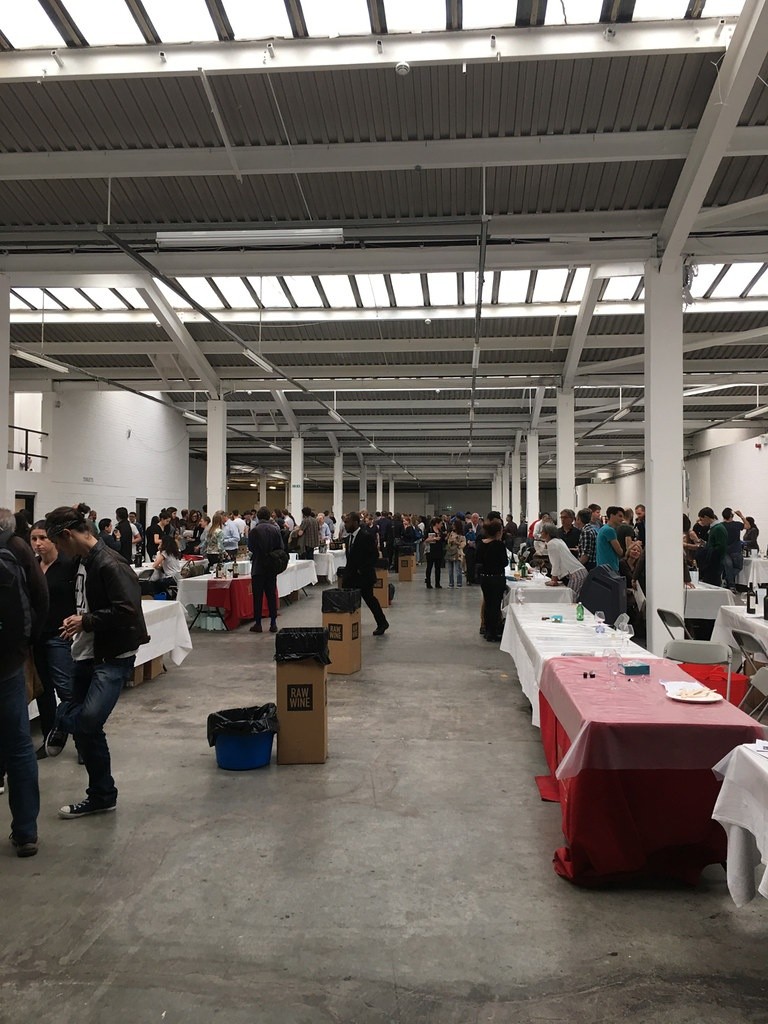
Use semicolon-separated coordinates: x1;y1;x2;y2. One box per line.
0;501;760;857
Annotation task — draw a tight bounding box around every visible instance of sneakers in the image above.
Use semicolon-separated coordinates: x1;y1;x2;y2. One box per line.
46;702;68;757
58;798;116;819
8;832;39;857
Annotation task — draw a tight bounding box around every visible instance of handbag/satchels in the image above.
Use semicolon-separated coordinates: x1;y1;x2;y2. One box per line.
268;550;288;575
180;559;205;578
445;545;458;561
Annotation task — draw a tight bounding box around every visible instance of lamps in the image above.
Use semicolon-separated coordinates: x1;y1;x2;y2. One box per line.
14;227;768;449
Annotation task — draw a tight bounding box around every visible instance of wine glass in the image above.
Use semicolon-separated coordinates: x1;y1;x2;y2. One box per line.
740;591;748;611
602;648;624;690
209;566;216;579
759;548;766;559
595;610;606;636
618;622;634;650
533;567;547;581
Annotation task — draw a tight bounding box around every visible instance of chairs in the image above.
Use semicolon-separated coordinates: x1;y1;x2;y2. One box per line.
657;609;768;724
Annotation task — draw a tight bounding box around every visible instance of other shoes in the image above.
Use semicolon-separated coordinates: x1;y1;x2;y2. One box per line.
34;743;48;760
484;634;502;642
269;626;277;632
250;624;262;632
373;620;389;635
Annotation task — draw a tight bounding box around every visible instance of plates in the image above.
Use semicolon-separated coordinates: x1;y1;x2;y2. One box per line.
666;688;723;703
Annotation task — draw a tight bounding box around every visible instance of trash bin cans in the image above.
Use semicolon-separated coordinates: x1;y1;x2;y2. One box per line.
275;627;329;764
399;546;412;582
321;588;363;675
212;707;275;772
404;542;417;573
372;558;390;608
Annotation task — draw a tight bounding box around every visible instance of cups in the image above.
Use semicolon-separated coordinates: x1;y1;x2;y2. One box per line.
751;549;758;559
152;555;157;561
290;553;296;563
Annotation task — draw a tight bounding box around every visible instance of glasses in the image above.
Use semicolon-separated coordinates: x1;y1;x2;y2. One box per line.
560;516;573;519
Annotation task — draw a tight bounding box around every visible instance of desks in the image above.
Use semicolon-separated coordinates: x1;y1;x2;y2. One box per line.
710;602;767;649
134;598;192;667
536;657;768;885
710;743;767;908
176;571;277;632
734;554;767;594
684;579;734;621
313;548;347;585
501;564;575;624
276;560;318;606
130;562;157;582
500;602;663;727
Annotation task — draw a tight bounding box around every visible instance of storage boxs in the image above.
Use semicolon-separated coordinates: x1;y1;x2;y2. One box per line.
275;658;329;764
322;609;361;674
144;657;166;680
399;554;416;582
374;570;389;609
126;665;143;687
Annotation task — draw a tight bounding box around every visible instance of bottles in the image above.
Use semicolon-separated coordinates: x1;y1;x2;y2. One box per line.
510;550;528;579
216;556;239;578
688;559;700;585
319;539;327;553
576;602;584;621
747;582;768;619
135;548;142;566
